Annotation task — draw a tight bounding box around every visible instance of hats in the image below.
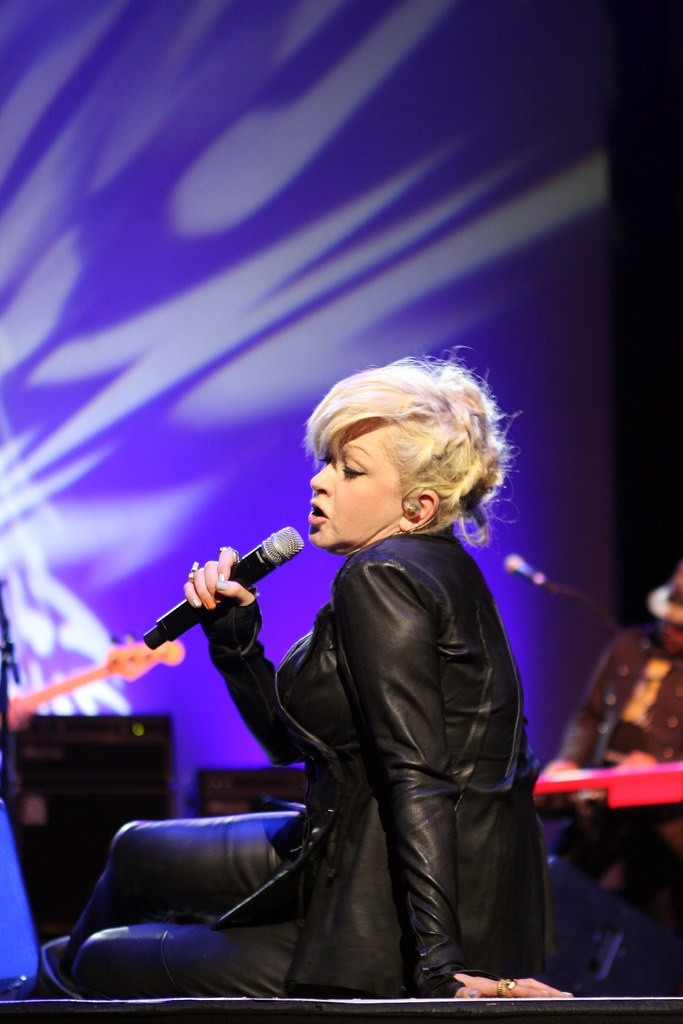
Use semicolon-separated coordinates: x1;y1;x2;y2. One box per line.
649;561;683;625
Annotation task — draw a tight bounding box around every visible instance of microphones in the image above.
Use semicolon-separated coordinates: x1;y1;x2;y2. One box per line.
142;527;304;650
502;554;549;588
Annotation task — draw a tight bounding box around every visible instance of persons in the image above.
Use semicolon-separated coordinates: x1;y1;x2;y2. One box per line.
540;567;683;922
4;352;585;1005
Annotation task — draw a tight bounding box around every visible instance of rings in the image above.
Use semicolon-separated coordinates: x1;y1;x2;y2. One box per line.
183;559;200;583
219;545;242;565
493;977;518;997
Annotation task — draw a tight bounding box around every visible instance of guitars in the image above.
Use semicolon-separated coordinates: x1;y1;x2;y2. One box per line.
7;635;189;737
